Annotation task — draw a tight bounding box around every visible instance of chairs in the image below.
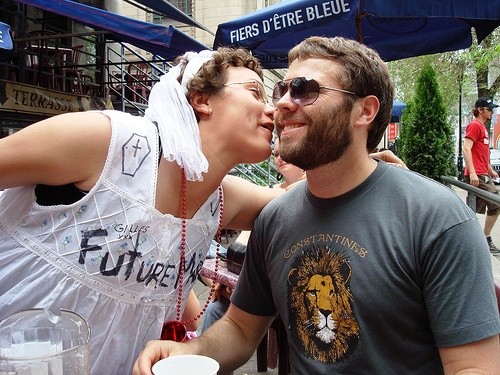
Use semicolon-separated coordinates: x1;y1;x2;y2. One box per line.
78;75;101;97
128;62;152;103
55;44;84;95
26;29;63;91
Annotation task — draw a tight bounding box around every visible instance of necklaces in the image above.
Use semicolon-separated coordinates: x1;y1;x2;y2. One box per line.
160;156;224;343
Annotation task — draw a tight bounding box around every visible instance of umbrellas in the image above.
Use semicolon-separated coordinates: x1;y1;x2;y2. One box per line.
212;0;500;71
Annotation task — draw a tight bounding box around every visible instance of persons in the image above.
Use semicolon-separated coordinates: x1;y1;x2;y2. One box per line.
131;36;500;375
180;273;216;332
0;46;409;375
463;97;500;255
201;137;306;374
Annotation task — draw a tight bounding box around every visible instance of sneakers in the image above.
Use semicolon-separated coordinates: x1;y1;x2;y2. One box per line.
485;236;500;254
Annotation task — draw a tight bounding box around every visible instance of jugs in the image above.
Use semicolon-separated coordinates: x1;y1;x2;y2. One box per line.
0;308;91;375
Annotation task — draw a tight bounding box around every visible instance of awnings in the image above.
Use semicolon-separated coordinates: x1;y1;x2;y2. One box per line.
2;0;214;62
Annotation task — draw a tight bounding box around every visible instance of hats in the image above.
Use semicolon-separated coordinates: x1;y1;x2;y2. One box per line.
474;96;500;108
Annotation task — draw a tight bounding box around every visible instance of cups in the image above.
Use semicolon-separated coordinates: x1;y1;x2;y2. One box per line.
152;355;220;375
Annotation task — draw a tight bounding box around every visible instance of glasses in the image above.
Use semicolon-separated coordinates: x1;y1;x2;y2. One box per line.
223;80;269;103
272;77;365;106
482;108;493;112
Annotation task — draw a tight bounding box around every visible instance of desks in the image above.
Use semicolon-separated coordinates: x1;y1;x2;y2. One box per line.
26;44;73;87
201;258;278;375
